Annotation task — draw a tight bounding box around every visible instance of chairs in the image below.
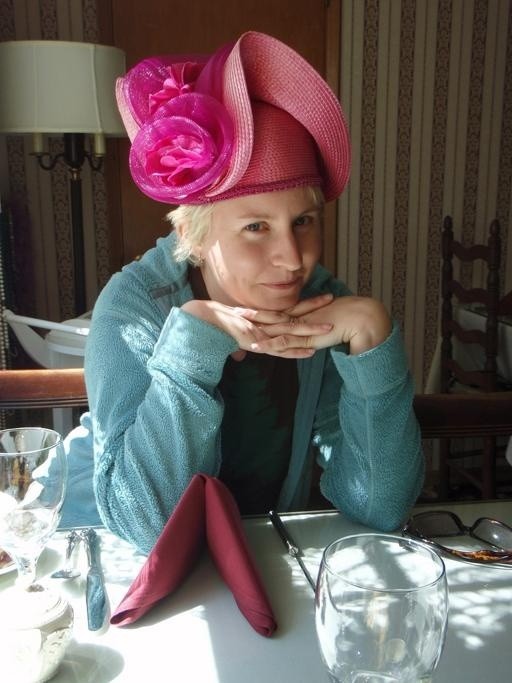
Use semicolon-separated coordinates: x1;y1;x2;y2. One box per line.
0;308;89;454
412;216;512;502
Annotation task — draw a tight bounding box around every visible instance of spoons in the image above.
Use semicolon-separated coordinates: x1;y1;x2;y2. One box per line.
49;527;82;579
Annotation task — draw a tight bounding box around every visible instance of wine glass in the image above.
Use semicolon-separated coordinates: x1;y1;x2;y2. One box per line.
0;427;66;619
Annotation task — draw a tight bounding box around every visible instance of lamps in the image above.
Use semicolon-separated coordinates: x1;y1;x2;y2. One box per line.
1;41;127;318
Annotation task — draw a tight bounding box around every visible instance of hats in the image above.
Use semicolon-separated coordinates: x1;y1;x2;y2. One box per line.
115;29;353;204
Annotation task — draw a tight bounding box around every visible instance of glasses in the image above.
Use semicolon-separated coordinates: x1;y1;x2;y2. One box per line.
401;505;512;563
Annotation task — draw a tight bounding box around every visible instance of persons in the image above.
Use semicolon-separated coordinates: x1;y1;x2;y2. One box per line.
22;29;432;557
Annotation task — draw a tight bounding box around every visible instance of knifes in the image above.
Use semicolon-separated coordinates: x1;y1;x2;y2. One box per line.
79;528;105;631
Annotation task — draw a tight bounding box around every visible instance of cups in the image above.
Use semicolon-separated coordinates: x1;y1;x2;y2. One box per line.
312;533;451;683
